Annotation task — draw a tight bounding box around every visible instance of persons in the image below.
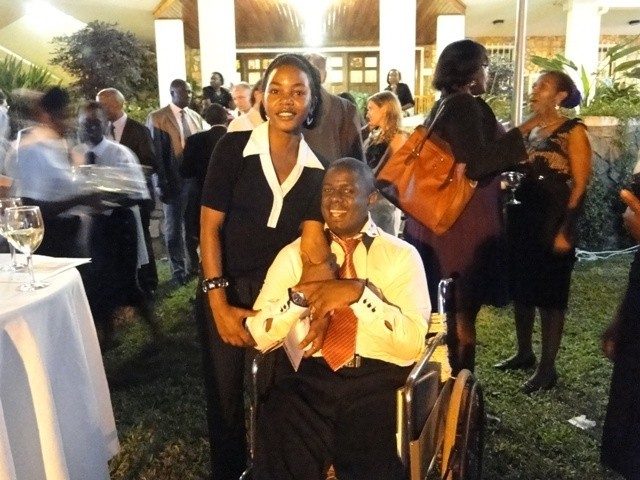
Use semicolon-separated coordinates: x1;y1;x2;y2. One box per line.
335;91;363;129
231;84;252;114
493;70;591;394
146;79;203;281
97;88;162;298
403;40;555;376
200;54;331;480
183;103;227;273
6;87;84;256
602;171;640;480
385;69;415;117
225;79;271;133
245;157;432;480
302;53;365;170
70;102;169;347
203;72;236;111
364;91;408;178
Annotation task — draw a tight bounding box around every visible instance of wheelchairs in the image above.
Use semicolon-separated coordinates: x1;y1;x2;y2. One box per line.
238;272;486;480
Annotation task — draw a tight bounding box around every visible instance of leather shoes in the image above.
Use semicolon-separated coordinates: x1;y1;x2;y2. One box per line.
519;372;557;394
492;355;536;371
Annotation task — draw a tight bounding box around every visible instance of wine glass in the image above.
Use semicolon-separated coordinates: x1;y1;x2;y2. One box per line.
1;198;21;279
5;206;44;291
502;170;525;208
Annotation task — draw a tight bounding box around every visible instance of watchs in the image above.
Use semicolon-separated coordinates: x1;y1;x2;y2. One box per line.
292;291;309;307
202;282;229;293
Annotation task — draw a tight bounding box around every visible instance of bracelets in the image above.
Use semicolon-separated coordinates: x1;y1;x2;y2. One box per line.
201;276;227;286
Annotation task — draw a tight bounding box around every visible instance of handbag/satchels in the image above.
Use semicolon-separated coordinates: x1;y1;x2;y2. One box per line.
374;91;479;235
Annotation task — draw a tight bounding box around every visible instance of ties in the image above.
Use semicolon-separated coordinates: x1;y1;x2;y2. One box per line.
179;110;192;141
109;121;115;140
85;152;97;164
321;230;362;373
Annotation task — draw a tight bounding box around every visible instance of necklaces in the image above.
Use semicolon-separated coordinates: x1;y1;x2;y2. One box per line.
535;122;560;133
